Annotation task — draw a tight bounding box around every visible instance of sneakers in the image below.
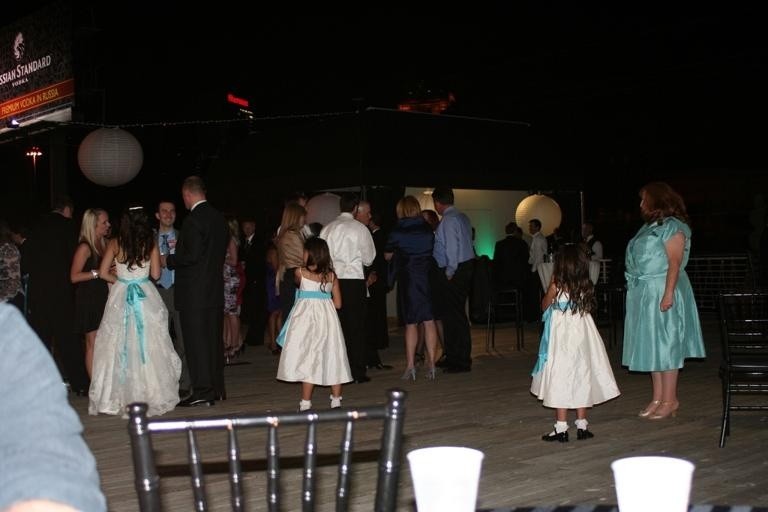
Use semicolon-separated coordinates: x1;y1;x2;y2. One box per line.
330;399;341;408
300;400;312;411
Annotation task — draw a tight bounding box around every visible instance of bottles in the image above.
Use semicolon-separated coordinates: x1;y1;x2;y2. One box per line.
161;235;170;256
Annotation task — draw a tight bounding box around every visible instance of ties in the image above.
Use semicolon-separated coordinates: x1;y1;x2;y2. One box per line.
160;235;172;289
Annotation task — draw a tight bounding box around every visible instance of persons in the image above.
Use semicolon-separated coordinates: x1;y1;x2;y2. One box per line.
527;218;548;323
264;187;475;411
515;228;522;239
1;175;264;420
494;222;529;287
620;181;707;420
0;305;108;510
581;223;603;285
530;243;621;442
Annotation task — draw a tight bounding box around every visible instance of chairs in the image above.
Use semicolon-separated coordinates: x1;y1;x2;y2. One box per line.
124;385;407;512
716;288;767;448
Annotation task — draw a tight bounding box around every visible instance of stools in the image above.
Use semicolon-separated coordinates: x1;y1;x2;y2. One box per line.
596;285;619;348
484;282;525;354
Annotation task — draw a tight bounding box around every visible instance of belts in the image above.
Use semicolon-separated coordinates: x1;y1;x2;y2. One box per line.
156;285;174;288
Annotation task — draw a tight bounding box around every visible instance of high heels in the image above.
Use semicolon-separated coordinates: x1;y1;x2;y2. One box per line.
638;400;661;417
354;351;471;382
648;401;679;420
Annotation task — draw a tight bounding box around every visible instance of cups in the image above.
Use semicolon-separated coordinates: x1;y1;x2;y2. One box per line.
548;253;553;263
612;456;695;512
406;446;484;512
543;254;549;263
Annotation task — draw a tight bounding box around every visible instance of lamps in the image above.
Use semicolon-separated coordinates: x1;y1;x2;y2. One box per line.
514;193;562;239
79;128;144;186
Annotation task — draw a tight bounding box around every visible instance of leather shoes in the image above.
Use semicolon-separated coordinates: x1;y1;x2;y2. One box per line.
577;429;594;440
180;391;226;407
542;429;568;442
